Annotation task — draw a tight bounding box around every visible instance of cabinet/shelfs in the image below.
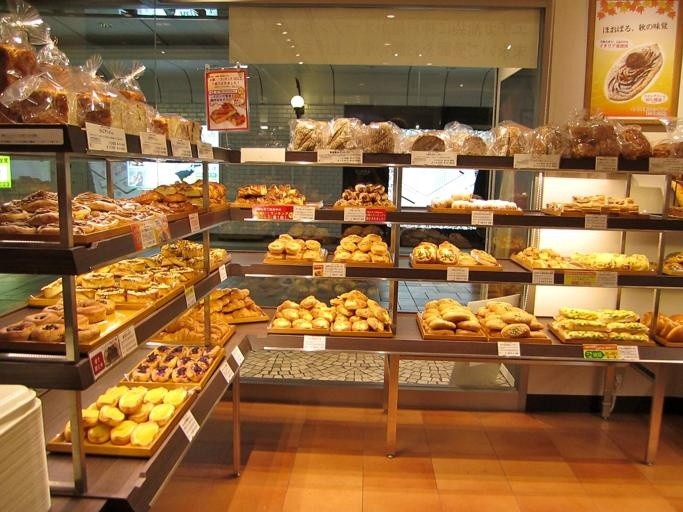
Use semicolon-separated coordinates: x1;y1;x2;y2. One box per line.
237;148;683;464
0;122;237;507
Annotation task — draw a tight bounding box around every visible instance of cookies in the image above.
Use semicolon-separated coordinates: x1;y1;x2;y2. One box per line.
606;42;663;102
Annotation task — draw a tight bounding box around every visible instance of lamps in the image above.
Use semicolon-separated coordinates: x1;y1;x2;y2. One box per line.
291;95;305;119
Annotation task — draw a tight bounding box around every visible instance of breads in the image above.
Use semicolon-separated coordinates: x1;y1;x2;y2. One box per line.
0;178;399;457
287;117;683;158
1;41;204;143
226;112;245;127
409;194;683;344
210;101;238;124
